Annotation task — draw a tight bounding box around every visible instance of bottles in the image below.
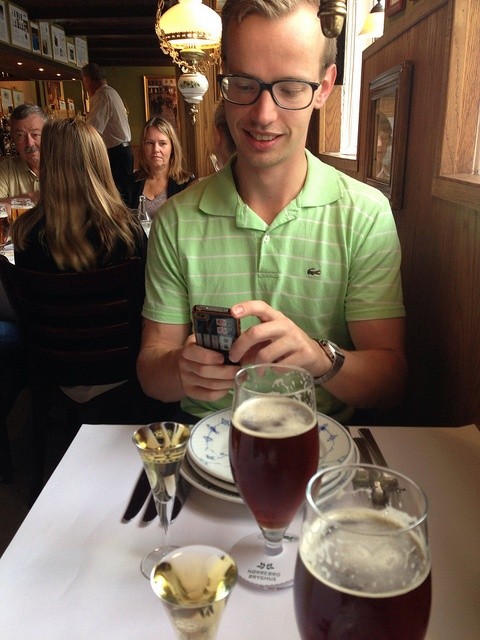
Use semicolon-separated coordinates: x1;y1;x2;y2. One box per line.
136;196;152;224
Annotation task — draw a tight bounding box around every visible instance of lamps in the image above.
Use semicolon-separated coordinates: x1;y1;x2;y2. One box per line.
357;0;385;38
154;0;222;123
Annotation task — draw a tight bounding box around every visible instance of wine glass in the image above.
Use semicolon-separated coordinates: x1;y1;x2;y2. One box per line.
292;462;433;640
150;544;238;639
225;362;320;590
131;421;192;581
0;202;10;255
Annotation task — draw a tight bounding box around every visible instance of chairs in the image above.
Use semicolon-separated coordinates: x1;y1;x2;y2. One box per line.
0;248;167;517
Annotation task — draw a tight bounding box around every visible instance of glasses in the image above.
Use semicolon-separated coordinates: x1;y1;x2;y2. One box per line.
215;67;322;111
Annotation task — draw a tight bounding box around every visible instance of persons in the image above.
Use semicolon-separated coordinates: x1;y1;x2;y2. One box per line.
119;116;196;220
82;63;133;180
0;103;49;199
135;0;410;426
10;117;148;425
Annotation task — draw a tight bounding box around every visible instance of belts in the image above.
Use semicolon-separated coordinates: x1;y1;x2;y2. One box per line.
119;141;131;148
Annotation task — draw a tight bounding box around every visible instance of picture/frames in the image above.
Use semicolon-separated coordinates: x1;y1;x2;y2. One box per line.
385;0;406;17
359;60;413;209
142;74;181;133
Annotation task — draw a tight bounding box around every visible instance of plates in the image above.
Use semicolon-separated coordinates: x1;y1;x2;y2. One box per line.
186;406;355;485
185;451;352;497
179;438;362;505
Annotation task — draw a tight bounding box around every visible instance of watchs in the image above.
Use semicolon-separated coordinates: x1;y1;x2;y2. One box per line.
313;338;345;385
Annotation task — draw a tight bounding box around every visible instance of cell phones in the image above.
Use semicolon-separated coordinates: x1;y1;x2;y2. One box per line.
190;305;242;365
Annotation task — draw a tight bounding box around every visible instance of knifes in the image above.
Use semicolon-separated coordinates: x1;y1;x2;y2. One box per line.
141;494;158;524
122;466;152;522
169;473;192;522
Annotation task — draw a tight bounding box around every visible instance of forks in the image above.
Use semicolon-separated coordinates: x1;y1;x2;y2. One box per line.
357;426;407;496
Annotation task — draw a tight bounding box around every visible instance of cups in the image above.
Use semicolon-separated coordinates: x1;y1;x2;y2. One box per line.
10;198;36;231
